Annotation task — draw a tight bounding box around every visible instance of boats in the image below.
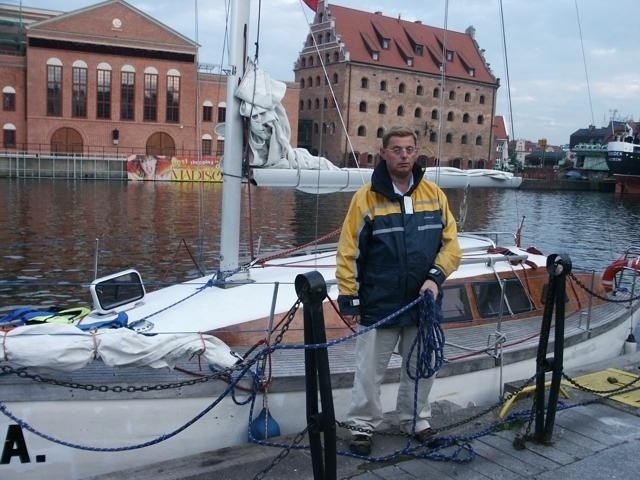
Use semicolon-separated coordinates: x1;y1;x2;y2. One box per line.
605;141;640;193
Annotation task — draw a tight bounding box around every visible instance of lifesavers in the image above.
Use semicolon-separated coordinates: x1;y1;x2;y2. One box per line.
602;258;640;293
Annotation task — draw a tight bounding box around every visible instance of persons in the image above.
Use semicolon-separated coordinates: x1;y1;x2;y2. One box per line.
334;127;460;455
132;154;164;180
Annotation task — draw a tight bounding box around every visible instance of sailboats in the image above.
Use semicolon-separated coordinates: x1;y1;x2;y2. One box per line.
0;0;639;480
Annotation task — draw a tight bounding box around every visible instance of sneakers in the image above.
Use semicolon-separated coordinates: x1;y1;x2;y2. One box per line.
414;429;444;448
350;434;371;455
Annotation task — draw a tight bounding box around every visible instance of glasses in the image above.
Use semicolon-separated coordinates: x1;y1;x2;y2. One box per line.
384;147;417;154
